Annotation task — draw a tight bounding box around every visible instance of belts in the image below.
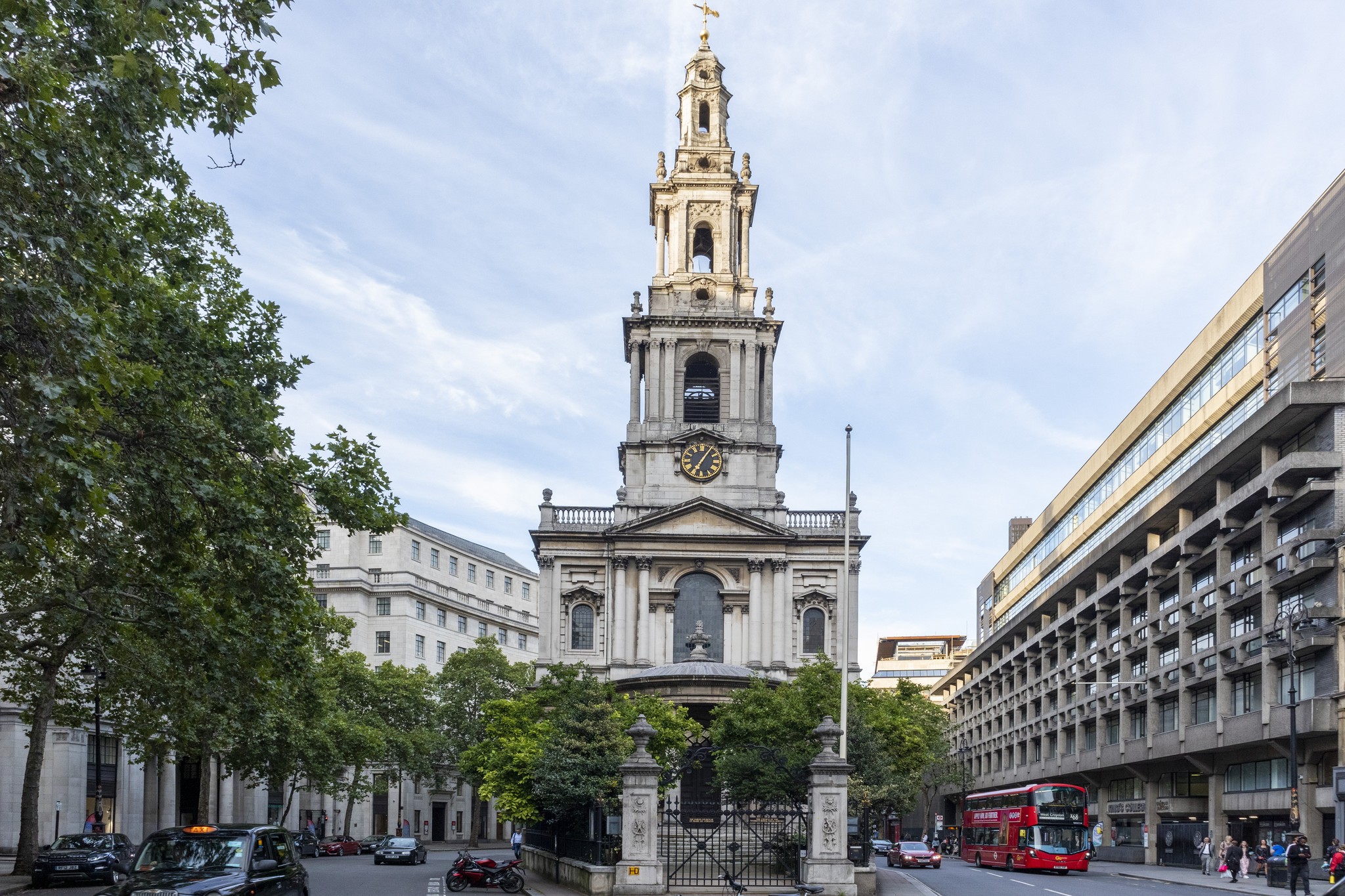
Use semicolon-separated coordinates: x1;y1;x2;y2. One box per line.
1202;853;1210;855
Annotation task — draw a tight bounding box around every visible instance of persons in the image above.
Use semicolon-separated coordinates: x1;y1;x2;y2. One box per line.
1239;841;1253;878
999;813;1008;844
1224;841;1243;883
1255;840;1270;878
900;834;904;842
1270;841;1286;856
1287;836;1314;896
943;835;958;855
905;832;911;841
511;828;521;860
1265;830;1273;847
1217;835;1233;872
967;831;998;845
1087;837;1095;865
273;818;280;826
1329;844;1345;880
921;833;928;842
932;837;939;853
1076;837;1084;846
1278;830;1288;851
1197;837;1217;876
268;818;272;825
1324;839;1339;883
875;830;879;839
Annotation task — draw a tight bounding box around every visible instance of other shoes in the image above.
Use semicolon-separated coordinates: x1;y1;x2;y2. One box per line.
1243;875;1249;878
1235;879;1238;882
1305;893;1314;896
1207;873;1210;875
1255;872;1259;878
1202;872;1206;875
1229;881;1236;883
1238;871;1242;878
1265;874;1268;879
1291;894;1296;896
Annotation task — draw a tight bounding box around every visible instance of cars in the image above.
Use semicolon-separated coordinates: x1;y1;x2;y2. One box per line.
29;833;138;886
359;834;396;854
374;837;427;866
886;842;941;869
320;835;362;856
870;839;893;857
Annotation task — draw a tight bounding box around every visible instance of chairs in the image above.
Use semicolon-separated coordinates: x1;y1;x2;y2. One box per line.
941;842;947;855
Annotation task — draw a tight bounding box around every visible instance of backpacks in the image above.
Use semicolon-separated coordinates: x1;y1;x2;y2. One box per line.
1336;852;1345;870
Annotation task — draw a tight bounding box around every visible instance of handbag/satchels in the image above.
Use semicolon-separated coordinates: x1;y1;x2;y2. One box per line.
1253;856;1258;861
1220;870;1233;879
1218;855;1225;858
1227;854;1234;862
1218;865;1228;873
512;844;515;850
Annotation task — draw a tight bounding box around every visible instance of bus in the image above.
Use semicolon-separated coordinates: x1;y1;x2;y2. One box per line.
960;783;1103;877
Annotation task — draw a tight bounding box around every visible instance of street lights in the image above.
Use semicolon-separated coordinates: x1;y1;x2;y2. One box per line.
956;738;972;820
1260;602;1319;841
80;654;102;830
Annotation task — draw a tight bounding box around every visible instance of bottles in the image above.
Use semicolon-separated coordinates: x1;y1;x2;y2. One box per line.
1330;875;1334;884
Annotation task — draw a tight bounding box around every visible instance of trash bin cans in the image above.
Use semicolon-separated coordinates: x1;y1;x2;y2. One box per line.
1267;856;1288;888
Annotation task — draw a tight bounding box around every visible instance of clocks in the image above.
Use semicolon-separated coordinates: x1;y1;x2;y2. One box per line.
680;443;723;482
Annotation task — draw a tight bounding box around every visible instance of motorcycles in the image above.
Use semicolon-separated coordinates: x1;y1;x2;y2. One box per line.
446;841;527;894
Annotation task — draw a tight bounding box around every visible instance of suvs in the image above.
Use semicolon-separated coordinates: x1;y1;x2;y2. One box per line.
91;822;312;896
289;830;321;858
